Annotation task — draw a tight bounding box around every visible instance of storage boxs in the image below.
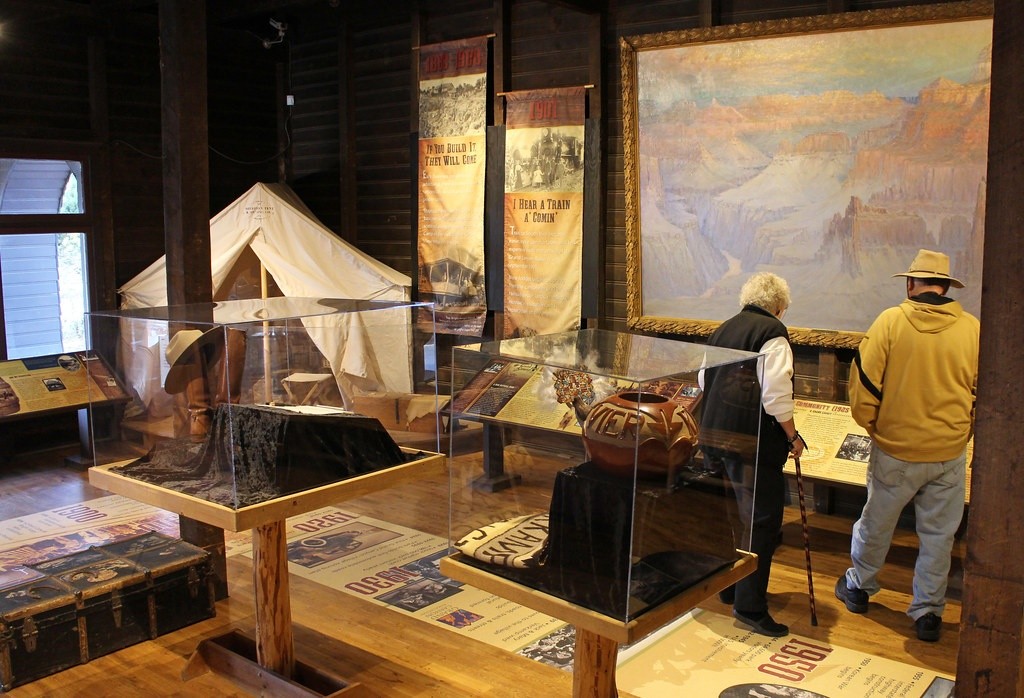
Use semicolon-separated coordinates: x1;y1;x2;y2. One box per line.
1;530;216;694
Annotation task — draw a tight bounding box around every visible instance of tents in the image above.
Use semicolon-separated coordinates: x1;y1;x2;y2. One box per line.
115;178;413;460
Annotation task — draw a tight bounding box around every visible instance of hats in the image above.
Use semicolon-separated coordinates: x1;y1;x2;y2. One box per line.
891;249;966;288
164;325;230;395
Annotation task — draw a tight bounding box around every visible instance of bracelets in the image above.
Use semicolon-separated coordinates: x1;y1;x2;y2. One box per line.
787;430;809;451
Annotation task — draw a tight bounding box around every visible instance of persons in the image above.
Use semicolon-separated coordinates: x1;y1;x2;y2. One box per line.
834;249;981;645
696;270;805;638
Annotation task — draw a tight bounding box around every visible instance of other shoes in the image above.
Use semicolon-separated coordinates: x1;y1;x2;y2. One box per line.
734;608;789;637
720;598;735;604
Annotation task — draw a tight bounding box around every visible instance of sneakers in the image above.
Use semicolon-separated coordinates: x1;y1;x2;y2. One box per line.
916;613;942;640
835;574;868;612
